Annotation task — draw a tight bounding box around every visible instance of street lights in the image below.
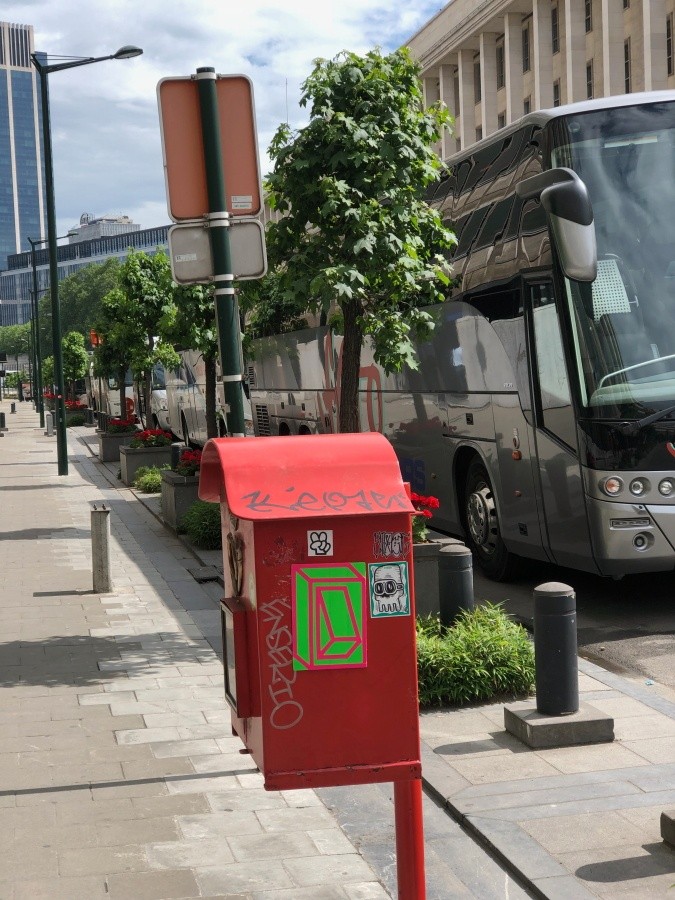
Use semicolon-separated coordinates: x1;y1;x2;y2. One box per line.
28;44;144;474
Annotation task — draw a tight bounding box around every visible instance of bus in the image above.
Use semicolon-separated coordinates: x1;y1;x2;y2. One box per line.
164;88;675;582
54;334;172;434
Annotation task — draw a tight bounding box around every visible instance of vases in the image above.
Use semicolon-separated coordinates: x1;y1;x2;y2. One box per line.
415;527;466;618
97;432;134;461
65;410;95;426
160;469;211;535
118;444;180;487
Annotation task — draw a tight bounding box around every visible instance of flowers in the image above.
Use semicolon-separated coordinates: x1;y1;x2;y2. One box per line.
179;448;203;468
406;491;437;542
134;429;172;446
63;400;89;409
109;417;135;428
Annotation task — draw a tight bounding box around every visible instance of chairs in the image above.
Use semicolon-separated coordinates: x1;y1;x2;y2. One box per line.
599;313;656;383
